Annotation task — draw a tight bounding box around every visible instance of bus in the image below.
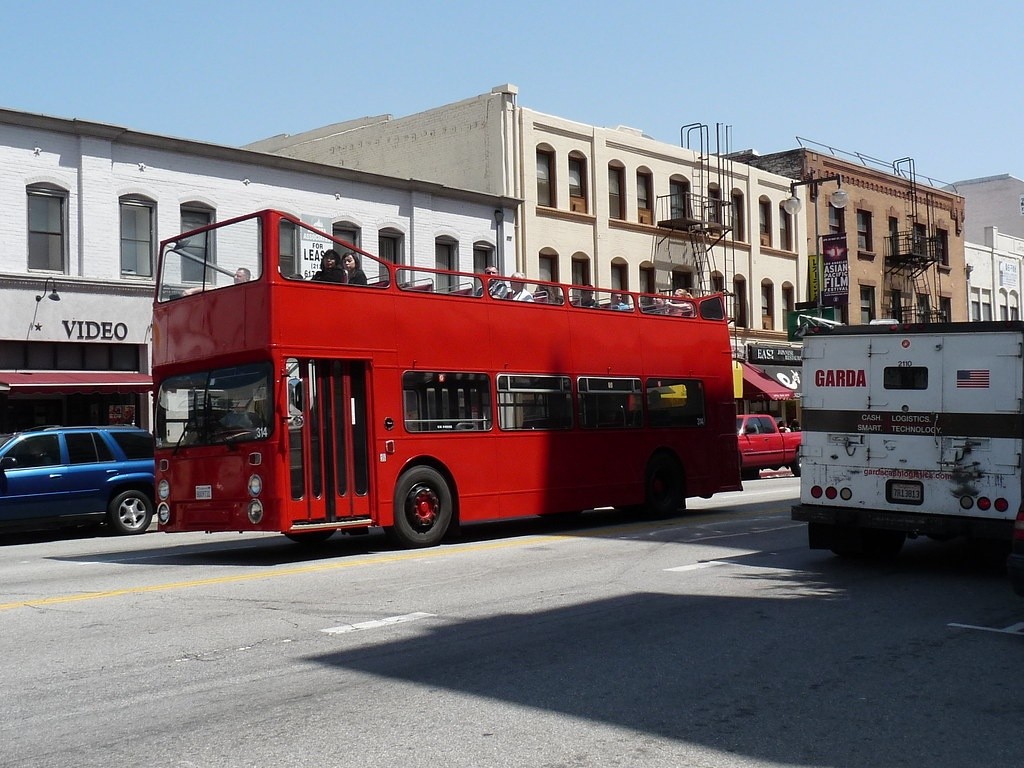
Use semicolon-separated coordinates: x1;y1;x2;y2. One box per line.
148;208;743;550
789;312;1024;572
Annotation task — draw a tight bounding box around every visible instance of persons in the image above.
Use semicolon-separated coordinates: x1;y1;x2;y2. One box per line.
536;284;563;304
289;250;343;283
183;268;251;296
653;288;695;316
778;420;785;432
474;267;507;299
341;252;368;285
605;293;629;309
573;284;600;307
218;397;253;434
510;273;532;301
791;419;800;431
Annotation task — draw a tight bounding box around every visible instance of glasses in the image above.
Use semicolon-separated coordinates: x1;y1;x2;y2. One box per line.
487;271;496;274
616;296;622;298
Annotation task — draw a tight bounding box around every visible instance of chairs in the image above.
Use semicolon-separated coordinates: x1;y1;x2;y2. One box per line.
455;422;478;430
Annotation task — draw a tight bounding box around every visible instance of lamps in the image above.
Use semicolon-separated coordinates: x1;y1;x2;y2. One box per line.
36;277;61;303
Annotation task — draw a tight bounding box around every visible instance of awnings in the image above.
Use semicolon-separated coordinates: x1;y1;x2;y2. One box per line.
647;386;674;393
0;373;153;394
739;361;802;400
659;361;743;399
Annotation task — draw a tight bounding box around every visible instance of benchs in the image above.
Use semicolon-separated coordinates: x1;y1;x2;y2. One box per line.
367;274;698;318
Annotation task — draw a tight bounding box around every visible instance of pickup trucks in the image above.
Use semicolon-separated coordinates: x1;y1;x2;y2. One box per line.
736;414;804;479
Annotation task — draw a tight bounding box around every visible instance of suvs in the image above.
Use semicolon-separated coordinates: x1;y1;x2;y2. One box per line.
0;422;164;536
